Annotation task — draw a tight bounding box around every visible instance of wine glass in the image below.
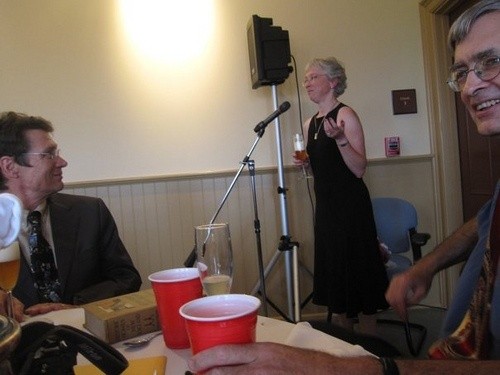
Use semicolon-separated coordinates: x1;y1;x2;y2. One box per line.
0;240;22;323
293;133;313;179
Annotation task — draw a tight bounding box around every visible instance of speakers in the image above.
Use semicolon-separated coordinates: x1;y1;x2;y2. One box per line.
245;14;293;88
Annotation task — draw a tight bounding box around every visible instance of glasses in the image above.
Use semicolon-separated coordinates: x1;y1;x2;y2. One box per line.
444;55;500;92
20;150;59;160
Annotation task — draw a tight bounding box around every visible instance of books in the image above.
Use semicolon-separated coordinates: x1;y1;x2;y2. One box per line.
82;289;162;344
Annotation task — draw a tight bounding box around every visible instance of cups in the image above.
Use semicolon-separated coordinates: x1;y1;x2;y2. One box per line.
148;268;206;349
194;224;234;296
179;293;262;374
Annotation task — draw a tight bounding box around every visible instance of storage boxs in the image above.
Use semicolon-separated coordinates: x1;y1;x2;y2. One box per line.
81;291;160;345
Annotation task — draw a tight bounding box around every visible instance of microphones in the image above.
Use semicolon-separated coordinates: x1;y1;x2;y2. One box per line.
254;102;290;133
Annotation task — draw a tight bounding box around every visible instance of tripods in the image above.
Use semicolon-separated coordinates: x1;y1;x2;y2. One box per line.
249;86;314;323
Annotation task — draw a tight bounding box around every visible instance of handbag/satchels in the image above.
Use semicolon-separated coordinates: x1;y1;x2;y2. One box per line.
9;321;129;375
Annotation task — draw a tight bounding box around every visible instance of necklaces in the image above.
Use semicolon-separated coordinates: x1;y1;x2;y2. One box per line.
314;100;338;139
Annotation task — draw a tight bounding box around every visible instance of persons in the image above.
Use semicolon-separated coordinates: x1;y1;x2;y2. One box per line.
291;58;392;335
0;110;142;321
187;0;500;375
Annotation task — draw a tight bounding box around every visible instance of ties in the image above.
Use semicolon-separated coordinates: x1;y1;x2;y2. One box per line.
27;211;63;303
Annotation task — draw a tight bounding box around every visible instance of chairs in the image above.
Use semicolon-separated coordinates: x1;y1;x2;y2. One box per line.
327;197;431;357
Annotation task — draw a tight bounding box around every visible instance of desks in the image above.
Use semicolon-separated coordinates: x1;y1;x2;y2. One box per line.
20;290;374;375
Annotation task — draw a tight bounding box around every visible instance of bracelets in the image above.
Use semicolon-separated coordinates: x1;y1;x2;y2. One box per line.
380;357;400;375
301;161;310;169
341;141;349;147
